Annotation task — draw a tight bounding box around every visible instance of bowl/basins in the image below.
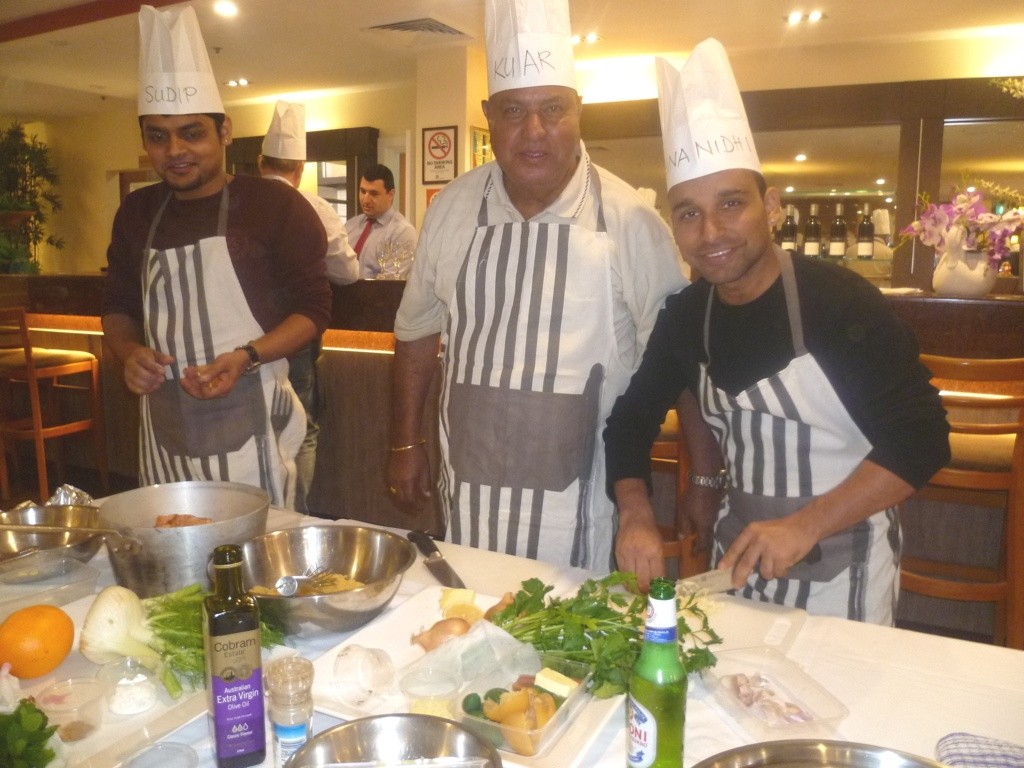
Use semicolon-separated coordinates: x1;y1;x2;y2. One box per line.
207;523;418;635
120;741;199;768
35;675;109;742
99;480;272;603
96;657;168;715
690;737;949;768
0;503;105;584
282;712;503;768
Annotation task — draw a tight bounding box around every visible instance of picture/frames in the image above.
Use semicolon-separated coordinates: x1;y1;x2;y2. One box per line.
422;125;459;185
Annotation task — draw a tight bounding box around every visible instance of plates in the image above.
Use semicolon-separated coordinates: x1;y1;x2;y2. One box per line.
301;583;507;726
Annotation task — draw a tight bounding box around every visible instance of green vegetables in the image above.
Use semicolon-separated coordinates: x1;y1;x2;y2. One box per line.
468;568;721;699
1;697;60;768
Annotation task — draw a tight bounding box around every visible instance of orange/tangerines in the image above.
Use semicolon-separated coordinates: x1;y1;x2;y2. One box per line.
0;602;74;679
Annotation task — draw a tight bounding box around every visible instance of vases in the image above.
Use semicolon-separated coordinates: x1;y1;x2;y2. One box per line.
931;250;997;297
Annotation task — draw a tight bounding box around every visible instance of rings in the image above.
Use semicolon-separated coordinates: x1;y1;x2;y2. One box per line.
390;486;399;493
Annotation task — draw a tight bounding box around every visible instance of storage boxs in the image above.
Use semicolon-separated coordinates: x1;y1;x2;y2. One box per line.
0;556;99;622
697;646;848;743
451;650;598;756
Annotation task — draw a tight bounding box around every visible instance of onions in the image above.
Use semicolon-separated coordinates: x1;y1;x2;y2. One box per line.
411;617;470;656
79;584;147;669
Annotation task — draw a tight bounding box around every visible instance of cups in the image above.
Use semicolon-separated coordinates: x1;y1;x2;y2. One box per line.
406;240;417;262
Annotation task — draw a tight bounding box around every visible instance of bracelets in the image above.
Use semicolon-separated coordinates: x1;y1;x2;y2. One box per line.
391;438;426;453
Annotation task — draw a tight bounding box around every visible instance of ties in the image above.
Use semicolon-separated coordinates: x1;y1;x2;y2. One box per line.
352;218;374;258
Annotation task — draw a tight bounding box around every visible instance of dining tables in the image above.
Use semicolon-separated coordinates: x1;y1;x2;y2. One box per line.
1;493;1024;768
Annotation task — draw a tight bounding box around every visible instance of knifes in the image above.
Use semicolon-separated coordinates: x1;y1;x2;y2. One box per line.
663;541;825;600
407;527;465;589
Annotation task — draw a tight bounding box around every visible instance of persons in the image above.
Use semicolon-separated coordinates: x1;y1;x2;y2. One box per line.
251;127;360;285
101;68;334;516
388;36;722;580
601;116;956;624
341;164;418;281
845;223;893;285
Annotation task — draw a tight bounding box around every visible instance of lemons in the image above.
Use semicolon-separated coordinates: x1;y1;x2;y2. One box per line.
463;685;565;757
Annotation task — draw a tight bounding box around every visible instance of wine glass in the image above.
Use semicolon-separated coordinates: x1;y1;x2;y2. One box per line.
375;240;392;281
391;241;407;280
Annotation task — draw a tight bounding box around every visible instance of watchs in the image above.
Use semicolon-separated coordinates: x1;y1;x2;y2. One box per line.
233;346;261;376
688;468;731;491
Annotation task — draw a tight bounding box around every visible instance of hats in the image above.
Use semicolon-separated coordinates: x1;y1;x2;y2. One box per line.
656;38;762;192
484;0;578;99
137;4;225;116
262;100;307;161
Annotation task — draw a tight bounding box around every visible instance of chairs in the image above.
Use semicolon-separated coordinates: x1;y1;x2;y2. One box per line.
895;354;1023;650
0;306;112;505
640;402;710;580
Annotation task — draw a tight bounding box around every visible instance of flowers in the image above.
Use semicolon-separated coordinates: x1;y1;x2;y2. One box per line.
888;174;1024;277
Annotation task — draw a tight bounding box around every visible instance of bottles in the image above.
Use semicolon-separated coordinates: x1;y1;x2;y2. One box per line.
778;204;797;251
830;202;847;260
201;549;267;767
805;203;822;260
857;202;874;260
628;584;687;768
264;657;314;768
